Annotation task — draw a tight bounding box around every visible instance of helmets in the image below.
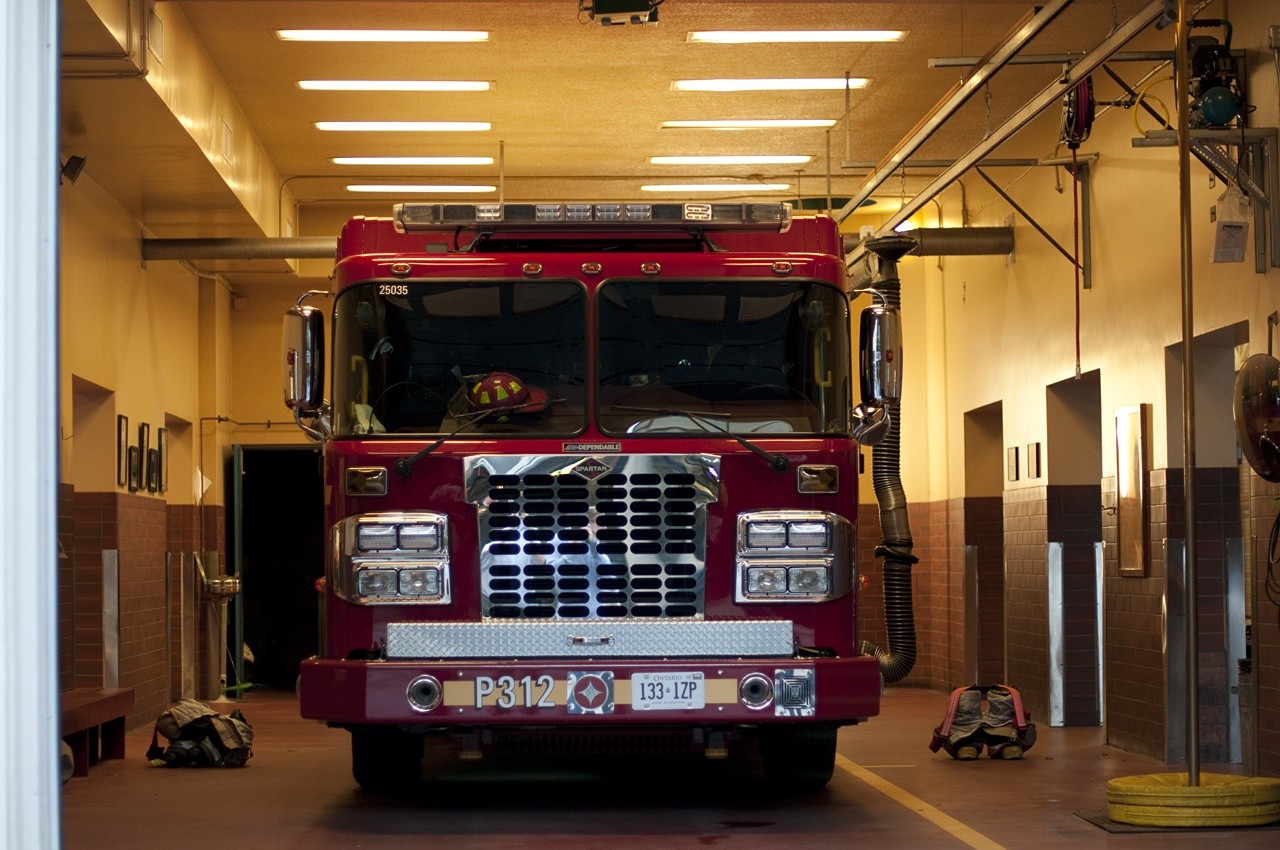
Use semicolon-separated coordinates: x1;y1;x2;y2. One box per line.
468;372;549;421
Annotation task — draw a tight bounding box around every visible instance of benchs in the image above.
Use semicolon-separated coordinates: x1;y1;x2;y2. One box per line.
60;688;137;777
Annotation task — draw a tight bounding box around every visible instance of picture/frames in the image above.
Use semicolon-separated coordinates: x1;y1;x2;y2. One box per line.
1028;442;1041;479
159;427;168;492
141;422;149;489
1115;402;1149;578
119;415;128;486
148;448;159;493
129;446;140;491
1008;447;1018;480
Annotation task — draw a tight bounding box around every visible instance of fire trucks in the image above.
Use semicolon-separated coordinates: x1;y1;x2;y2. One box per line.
283;199;905;808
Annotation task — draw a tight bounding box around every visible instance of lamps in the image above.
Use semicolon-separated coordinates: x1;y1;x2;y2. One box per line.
58;154;87;185
